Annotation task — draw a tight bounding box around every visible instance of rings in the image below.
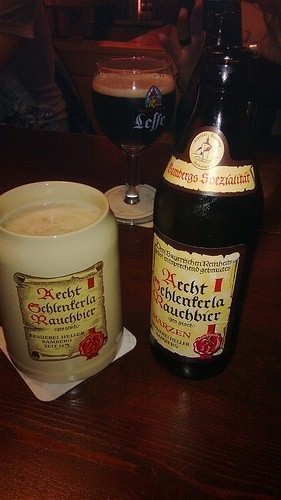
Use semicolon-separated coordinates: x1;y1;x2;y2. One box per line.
178;34;193;46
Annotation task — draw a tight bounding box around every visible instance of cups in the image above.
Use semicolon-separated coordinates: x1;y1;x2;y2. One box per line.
0;181;123;383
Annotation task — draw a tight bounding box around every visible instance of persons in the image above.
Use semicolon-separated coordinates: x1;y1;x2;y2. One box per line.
0;1;69;131
158;0;280;152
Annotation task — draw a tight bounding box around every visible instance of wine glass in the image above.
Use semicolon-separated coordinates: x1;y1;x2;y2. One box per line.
89;54;176;226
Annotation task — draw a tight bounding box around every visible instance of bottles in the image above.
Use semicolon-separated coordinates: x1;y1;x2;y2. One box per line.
147;45;263;381
186;1;245;85
